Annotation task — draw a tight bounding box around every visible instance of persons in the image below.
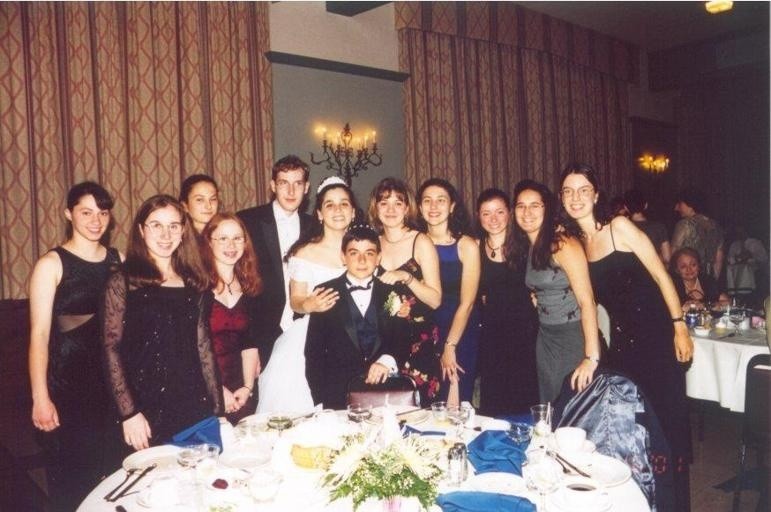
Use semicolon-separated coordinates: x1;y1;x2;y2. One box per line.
256;174;365;420
474;188;539;415
504;179;600;429
558;162;696;510
303;224;413;413
28;182;126;511
103;194;224;468
181;173;265;424
416;178;482;411
610;195;769;325
368;177;444;411
232;154;322;368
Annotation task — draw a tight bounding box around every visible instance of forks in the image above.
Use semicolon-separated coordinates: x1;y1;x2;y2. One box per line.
103;465;140;500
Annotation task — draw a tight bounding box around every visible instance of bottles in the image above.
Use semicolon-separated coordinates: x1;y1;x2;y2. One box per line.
447;442;469;484
687;298;751;331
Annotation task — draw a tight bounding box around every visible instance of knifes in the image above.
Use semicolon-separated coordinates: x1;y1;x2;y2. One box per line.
110;461;161;502
556;451;592;479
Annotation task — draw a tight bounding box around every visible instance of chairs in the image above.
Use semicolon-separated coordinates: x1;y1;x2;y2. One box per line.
723;350;771;509
346;375;425;416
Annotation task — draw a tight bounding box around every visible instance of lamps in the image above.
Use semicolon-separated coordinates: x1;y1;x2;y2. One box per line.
309;119;385;196
636;152;674;186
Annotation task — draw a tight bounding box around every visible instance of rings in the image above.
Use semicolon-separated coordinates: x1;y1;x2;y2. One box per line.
327;302;330;305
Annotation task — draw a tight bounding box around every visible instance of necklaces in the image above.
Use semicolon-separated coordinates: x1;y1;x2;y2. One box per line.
486;237;504;258
384;231;407;243
220;277;234;296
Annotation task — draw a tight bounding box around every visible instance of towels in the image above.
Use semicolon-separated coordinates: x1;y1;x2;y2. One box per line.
462;420;527;481
431;487;542;511
160;411;225;454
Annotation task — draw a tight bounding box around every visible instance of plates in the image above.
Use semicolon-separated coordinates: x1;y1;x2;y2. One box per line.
572;438;596;456
479;417;511;432
473;471;531;496
123;443;191;478
219;446;273;469
369;404;430;426
582;454;632;487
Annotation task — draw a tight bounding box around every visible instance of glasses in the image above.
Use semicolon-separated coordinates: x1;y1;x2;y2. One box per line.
560;187;595;197
144;222;183;234
213;236;243;244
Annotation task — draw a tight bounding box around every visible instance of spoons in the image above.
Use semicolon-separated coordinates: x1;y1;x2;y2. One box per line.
550;454;573;476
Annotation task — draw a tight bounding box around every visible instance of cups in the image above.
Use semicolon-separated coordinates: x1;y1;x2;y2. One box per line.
530;404;555;432
565;483;613;512
346;401;374;421
430;401;471;434
555;426;587;451
758;321;767;336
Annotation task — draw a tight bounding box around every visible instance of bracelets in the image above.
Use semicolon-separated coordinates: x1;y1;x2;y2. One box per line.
402;272;413;284
446;340;456;347
585;356;600;361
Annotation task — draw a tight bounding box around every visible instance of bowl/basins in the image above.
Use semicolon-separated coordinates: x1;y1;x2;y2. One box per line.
694;325;712;336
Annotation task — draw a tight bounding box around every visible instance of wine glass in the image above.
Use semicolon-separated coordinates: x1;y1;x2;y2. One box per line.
505;423;533;467
180;444;221;487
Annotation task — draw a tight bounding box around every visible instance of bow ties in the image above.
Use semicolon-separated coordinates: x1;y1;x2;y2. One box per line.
349;285;369;292
